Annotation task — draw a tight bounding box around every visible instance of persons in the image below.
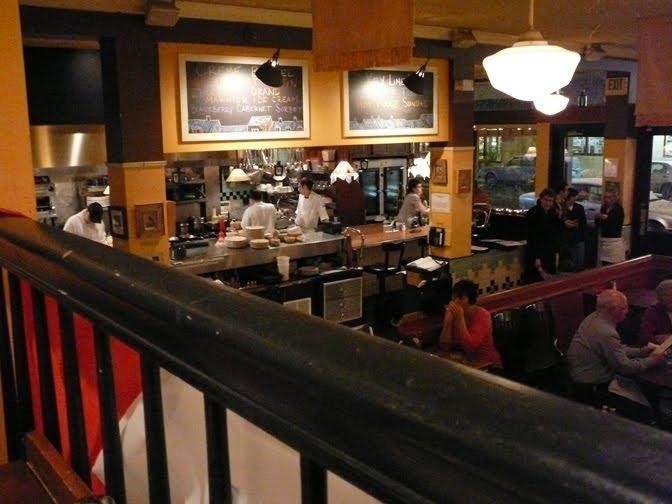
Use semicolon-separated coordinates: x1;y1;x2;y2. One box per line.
566;289;668;422
397;179;430;259
523;183;624;285
294;177;329;229
241;190;277;235
438;280;503;374
640;280;672;347
473;180;491;220
63;202;106;244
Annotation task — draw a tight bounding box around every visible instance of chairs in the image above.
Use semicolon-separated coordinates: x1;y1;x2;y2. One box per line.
362;238;409;318
426;294;671;432
404;202;497;280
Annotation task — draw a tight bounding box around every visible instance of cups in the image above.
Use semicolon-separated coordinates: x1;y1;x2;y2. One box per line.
178;217;206;236
275;253;290;282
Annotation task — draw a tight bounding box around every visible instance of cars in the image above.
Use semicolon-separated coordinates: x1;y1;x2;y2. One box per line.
650;162;672;197
365;157;430;198
519;177;671;234
480;151;580;190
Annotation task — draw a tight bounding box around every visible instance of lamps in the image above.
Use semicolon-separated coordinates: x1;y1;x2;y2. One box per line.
325;146;361;185
407;154;430;182
402;35;433;96
531;84;570;117
222;150;252;182
253;34;285;88
479;1;582;103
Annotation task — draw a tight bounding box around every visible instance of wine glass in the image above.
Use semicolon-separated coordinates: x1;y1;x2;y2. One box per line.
242;147;313;174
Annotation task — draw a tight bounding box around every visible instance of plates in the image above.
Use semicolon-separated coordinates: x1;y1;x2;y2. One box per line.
225;224;270;250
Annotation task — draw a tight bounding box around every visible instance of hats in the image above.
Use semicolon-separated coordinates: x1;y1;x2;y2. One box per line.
88;202;104;223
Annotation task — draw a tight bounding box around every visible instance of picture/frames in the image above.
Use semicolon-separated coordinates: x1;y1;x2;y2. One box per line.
455;168;472;194
134;202;166;238
431;159;448;186
107;205;130;240
